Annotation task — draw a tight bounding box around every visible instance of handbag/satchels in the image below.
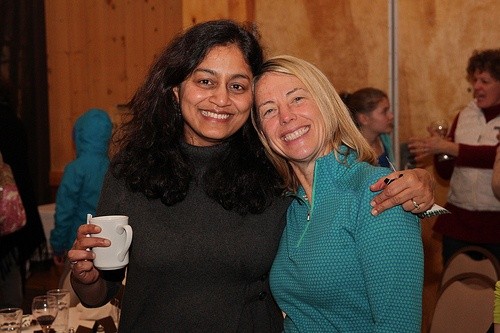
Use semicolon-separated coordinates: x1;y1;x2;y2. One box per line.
0;151;27;236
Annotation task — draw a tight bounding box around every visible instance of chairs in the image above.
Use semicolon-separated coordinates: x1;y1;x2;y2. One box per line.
58;267;80;307
426;246;500;333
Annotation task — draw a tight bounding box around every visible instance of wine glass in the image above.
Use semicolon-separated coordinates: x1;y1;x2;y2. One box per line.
430;119;453;161
32;295;59;333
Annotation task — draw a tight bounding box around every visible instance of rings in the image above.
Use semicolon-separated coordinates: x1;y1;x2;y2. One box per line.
411;198;419;208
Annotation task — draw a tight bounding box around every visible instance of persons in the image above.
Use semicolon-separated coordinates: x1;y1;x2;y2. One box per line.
336;87;400;168
247;55;425;333
49;107;114;290
0;38;47;310
407;46;500;274
68;17;437;333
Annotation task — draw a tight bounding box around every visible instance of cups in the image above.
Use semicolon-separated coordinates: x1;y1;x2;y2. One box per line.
0;308;23;333
47;288;70;333
89;216;133;270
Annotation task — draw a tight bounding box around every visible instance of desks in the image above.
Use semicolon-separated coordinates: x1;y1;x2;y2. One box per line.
38;201;65;272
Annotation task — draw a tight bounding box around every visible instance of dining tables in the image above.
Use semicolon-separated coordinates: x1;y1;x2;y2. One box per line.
0;305;122;333
